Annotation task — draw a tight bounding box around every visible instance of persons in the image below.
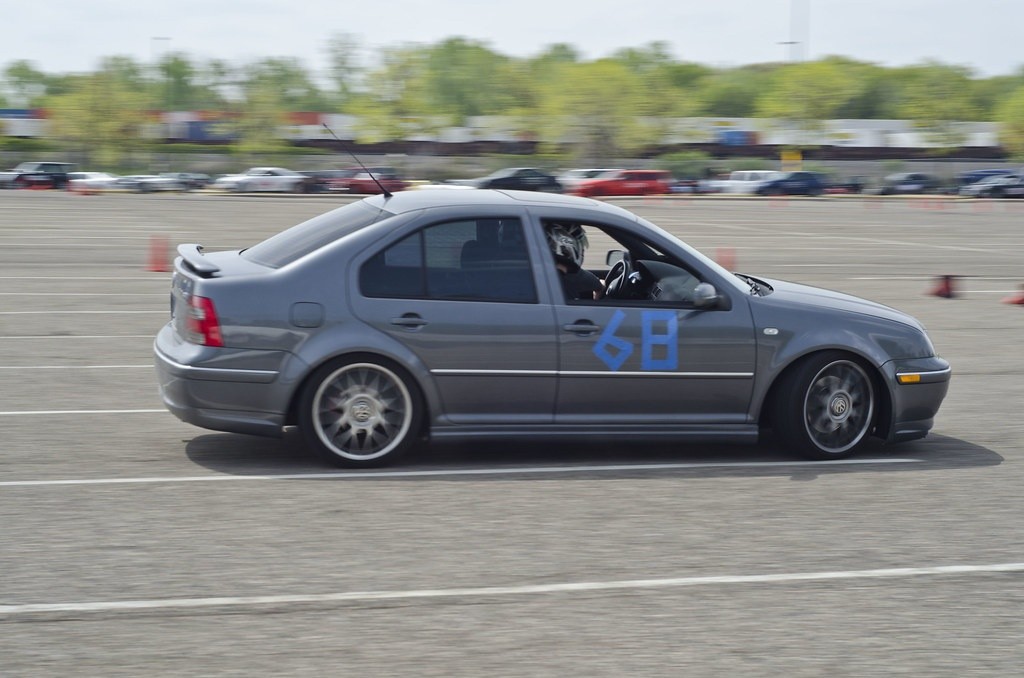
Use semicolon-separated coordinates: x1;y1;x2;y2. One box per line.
499;220;607;303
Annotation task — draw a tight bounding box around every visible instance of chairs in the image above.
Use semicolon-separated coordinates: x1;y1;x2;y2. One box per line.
460;241;537;304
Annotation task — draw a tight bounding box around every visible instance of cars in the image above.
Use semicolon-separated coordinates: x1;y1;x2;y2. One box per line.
153;185;952;470
1;160;1024;197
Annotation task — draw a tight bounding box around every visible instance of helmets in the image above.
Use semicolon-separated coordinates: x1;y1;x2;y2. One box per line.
543;220;588;273
